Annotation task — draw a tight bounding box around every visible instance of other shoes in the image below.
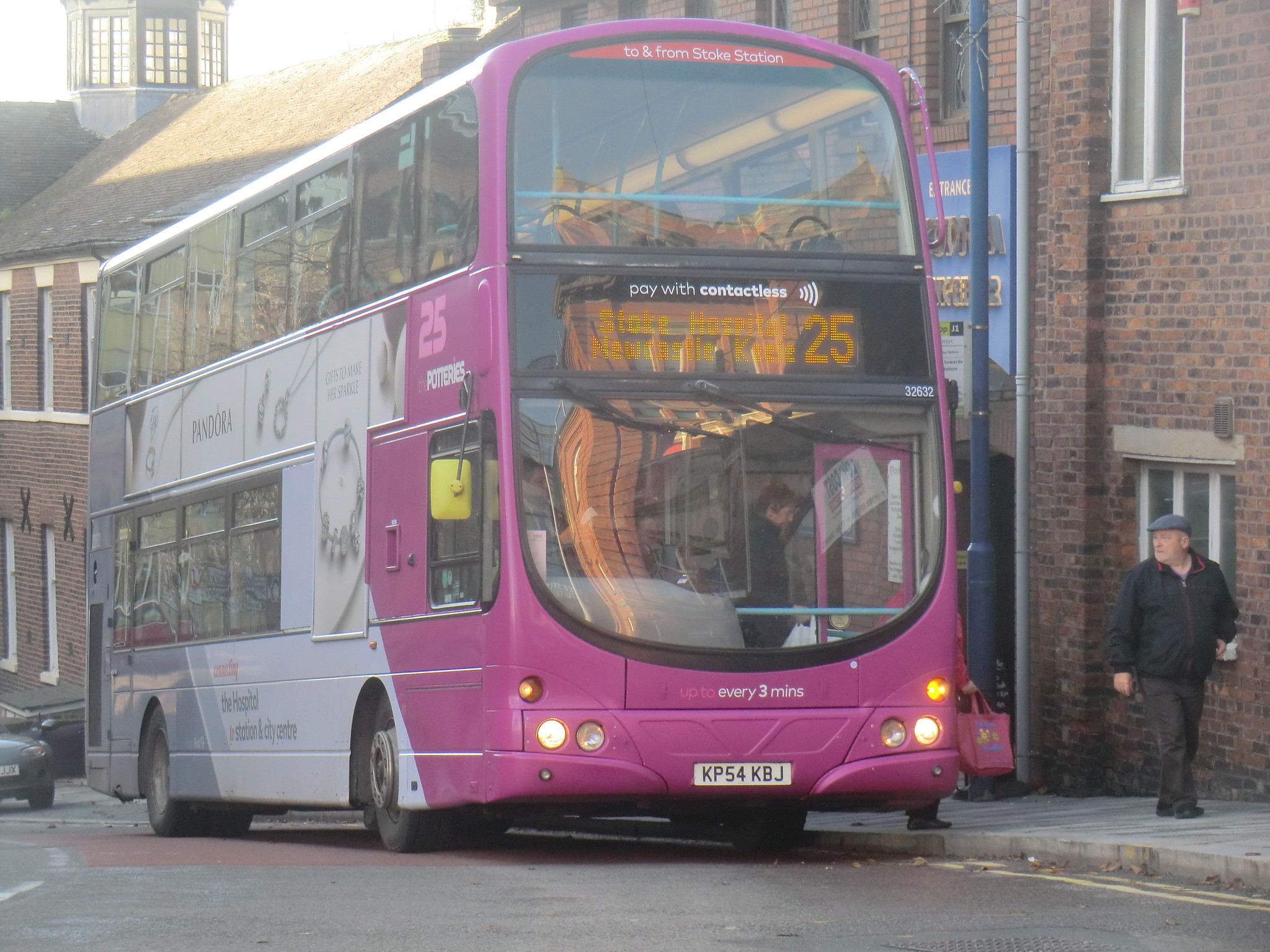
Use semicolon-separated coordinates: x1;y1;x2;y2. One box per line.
1184;807;1204;819
1156;801;1174;817
908;815;952;830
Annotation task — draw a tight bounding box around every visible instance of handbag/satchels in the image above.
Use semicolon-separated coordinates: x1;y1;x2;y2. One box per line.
782;615;817;648
958;689;1014;776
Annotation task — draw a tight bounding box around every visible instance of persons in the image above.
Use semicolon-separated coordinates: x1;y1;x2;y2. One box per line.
724;485;811;642
877;581;978;830
1109;514;1238;818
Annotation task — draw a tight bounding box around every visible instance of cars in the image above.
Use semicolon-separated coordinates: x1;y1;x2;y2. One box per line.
0;732;57;810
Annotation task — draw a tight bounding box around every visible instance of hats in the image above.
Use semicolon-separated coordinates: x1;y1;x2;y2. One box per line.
1147;514;1192;538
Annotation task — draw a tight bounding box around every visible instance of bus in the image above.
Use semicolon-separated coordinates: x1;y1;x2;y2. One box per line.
84;17;972;855
